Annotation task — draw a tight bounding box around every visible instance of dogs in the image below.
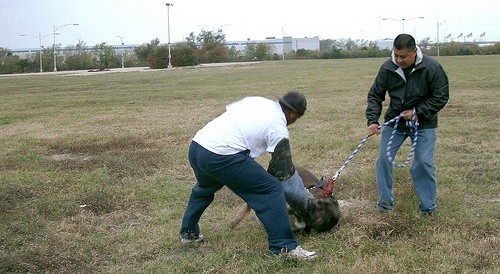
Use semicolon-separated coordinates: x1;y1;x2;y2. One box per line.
229;163;340;232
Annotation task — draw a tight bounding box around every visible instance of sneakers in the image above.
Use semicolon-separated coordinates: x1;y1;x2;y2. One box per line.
285;246;318;262
179;233;204;244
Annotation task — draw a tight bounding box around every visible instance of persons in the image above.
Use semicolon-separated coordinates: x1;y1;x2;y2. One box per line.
365;33;449;218
179;90;317;262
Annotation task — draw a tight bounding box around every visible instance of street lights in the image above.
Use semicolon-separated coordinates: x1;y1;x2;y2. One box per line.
437;20;447;56
165;3;173;70
19;30;60;73
53;24;80;72
381;15;424;34
116;35;124;68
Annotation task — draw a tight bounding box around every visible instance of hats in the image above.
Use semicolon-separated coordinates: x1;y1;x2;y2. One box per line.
278;91;307;115
